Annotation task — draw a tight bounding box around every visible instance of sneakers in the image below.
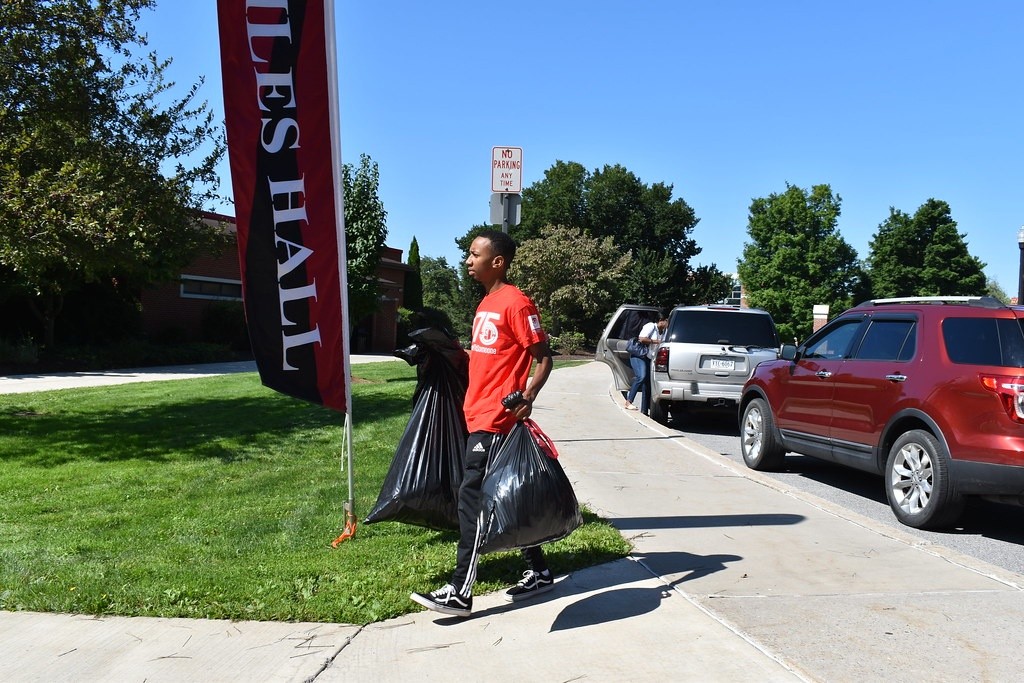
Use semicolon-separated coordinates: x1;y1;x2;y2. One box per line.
504;569;554;601
409;583;474;617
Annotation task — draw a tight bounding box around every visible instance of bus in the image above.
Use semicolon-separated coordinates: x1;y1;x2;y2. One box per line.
723;284;741;304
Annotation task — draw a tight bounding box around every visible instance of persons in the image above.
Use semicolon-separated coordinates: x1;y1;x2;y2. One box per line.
625;311;670;417
410;229;553;617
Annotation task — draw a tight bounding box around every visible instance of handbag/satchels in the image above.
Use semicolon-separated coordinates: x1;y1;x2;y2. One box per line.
625;327;655;359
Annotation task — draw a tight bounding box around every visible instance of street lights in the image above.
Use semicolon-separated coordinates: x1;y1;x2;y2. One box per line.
1015;225;1024;305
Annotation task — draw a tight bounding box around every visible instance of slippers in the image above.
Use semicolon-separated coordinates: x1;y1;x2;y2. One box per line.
625;404;638;410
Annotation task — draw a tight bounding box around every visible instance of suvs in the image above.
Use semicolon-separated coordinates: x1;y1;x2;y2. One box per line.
595;302;780;426
735;296;1024;531
